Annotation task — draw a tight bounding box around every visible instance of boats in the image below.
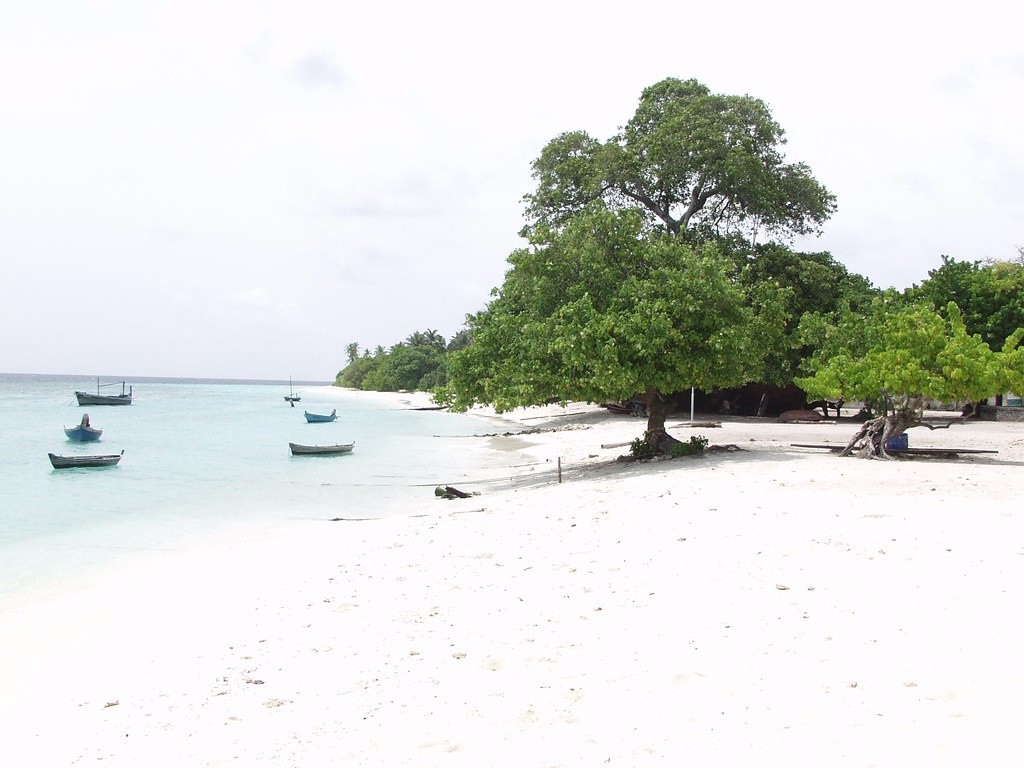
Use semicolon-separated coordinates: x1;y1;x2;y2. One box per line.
284;374;301;402
288;442;357;454
75;376;133;406
62;414;103;441
48;451;126;468
305;409;338;422
